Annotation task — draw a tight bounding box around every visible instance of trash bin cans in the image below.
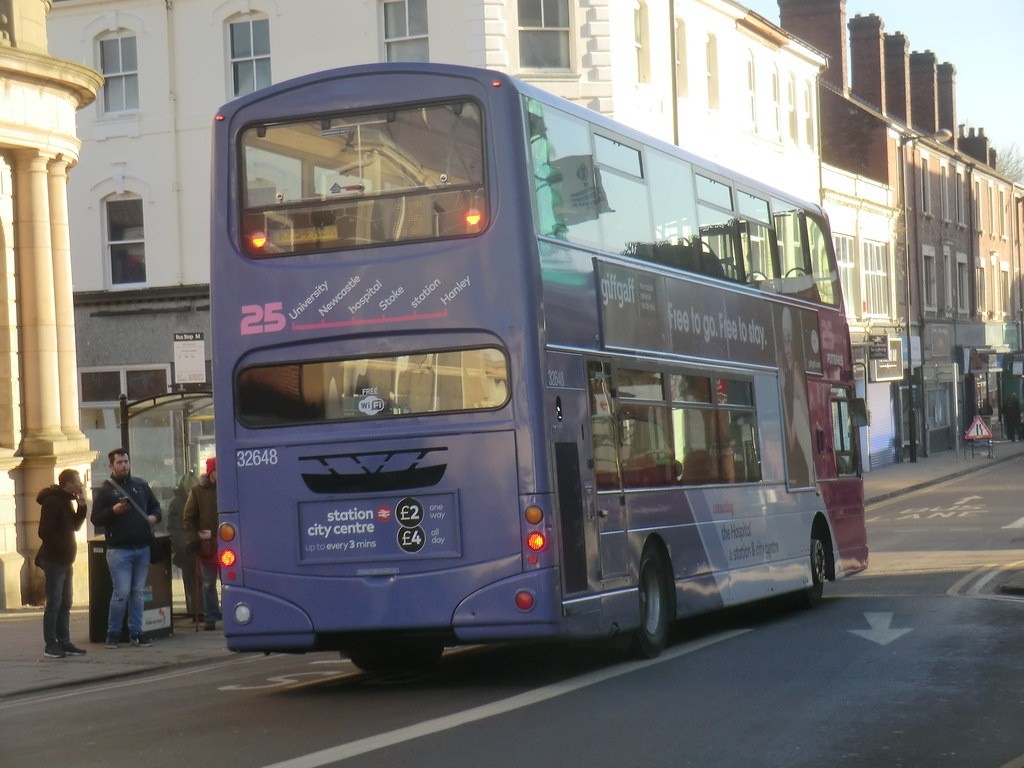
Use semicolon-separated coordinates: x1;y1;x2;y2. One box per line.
87;530;173;643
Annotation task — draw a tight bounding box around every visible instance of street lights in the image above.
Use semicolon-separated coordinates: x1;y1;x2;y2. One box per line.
900;128;952;462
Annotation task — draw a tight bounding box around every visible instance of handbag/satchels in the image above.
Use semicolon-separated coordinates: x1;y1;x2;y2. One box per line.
150;532;161;564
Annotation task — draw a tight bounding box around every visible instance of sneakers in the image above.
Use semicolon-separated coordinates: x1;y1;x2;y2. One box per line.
130;634;153;647
106;634;118;648
59;641;86;656
45;645;65;657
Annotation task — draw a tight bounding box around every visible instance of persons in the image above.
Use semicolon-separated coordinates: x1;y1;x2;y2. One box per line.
35;469;87;657
90;448;161;648
1003;393;1024;443
167;457;221;631
983;399;993;415
778;307;815;488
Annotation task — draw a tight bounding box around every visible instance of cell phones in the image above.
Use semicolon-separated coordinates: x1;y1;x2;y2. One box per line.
119;496;128;504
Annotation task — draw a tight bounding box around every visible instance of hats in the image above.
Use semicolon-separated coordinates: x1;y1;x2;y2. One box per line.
206;458;215;475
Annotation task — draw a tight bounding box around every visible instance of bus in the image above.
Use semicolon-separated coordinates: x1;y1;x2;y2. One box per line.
210;62;870;679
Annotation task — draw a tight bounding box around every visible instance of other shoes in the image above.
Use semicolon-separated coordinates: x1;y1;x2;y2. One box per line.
204;620;216;630
